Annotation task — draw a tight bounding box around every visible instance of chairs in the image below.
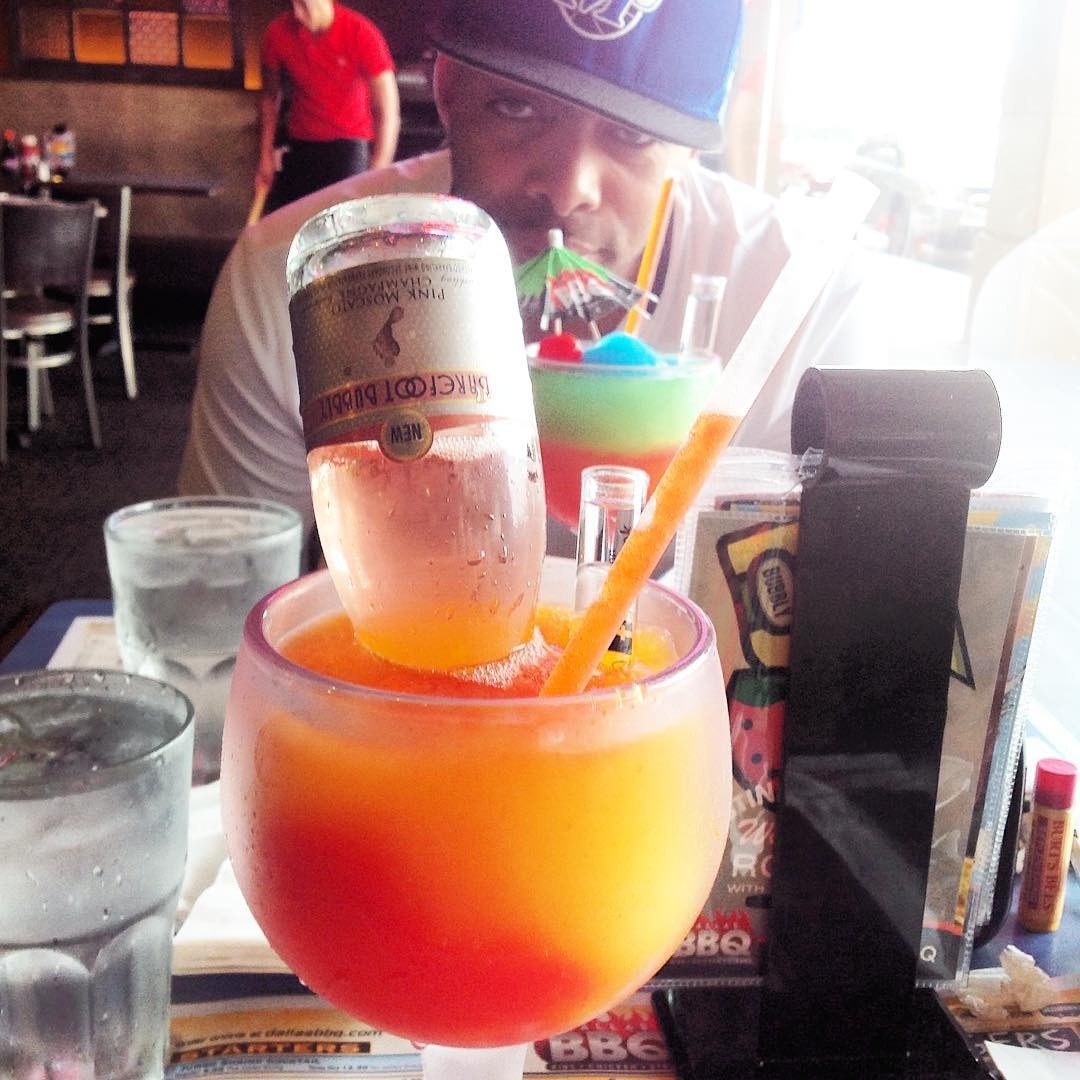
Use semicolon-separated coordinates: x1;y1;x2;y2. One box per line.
23;190;139;420
0;194;104;466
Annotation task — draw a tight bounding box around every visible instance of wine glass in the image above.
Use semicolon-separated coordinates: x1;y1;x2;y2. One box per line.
219;553;733;1080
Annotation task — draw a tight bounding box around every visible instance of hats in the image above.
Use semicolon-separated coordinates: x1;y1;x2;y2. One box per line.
426;0;744;151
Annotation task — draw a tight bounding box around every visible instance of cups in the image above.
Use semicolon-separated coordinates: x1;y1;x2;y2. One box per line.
103;495;302;788
526;337;720;589
1;664;195;1080
672;445;802;599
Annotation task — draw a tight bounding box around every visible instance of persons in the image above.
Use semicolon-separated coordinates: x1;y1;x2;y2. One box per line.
250;0;400;216
179;0;821;576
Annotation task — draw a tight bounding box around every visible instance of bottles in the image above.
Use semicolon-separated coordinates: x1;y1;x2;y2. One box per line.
287;193;546;677
574;466;650;674
1;121;77;180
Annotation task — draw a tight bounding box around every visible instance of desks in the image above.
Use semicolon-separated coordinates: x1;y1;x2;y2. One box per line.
1;154;216;203
